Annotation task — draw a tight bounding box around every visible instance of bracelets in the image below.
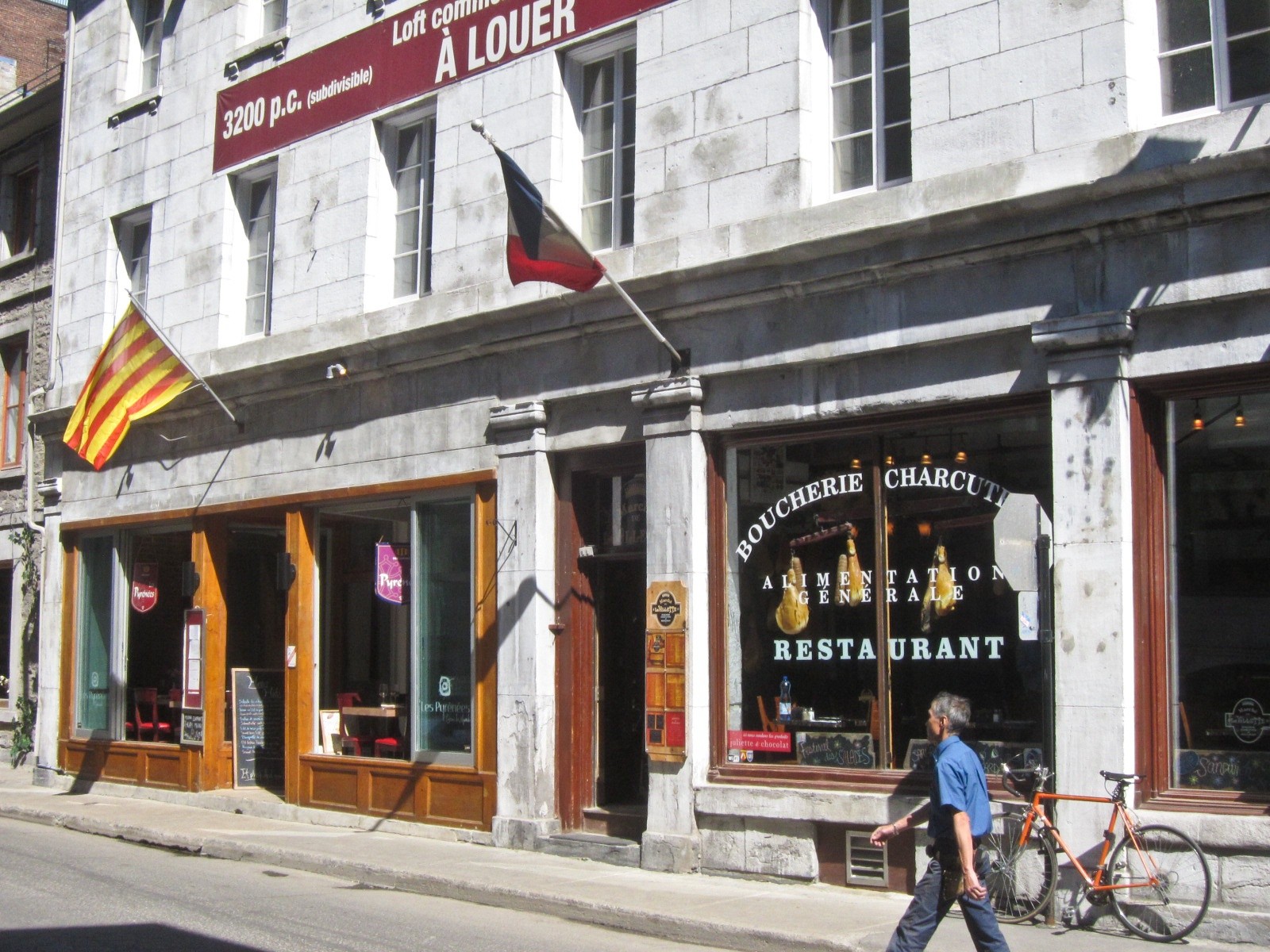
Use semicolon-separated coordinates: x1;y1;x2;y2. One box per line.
892;823;899;835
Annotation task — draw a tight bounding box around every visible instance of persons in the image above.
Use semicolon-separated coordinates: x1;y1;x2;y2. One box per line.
871;692;1009;951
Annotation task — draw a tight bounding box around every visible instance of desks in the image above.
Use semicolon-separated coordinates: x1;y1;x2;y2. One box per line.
155;693;183;742
342;706;404;737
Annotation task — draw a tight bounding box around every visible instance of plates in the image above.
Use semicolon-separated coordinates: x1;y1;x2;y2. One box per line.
380;703;405;709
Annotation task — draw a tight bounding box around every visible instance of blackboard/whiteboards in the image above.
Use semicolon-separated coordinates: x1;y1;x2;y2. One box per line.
180;712;205;746
1179;749;1269;795
903;739;976;771
795;731;876;770
975;741;1044;776
231;667;285;791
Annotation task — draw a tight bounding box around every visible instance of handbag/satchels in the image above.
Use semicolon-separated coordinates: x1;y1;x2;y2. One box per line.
942;848;977;901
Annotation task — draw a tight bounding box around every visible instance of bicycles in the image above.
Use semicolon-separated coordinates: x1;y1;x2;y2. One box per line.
973;760;1212;942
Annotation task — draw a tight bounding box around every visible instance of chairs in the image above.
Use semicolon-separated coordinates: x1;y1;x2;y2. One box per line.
373;736;406;760
337;693;376;758
128;686;171;744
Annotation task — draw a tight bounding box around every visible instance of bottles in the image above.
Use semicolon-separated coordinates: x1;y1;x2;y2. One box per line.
802;707;815;721
778;676;792;722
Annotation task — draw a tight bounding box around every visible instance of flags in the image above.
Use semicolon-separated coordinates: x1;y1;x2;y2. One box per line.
62;303;195;469
494;147;604;292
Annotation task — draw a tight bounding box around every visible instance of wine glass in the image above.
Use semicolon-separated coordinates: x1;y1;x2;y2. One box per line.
379;683;388;703
390;683;400;705
